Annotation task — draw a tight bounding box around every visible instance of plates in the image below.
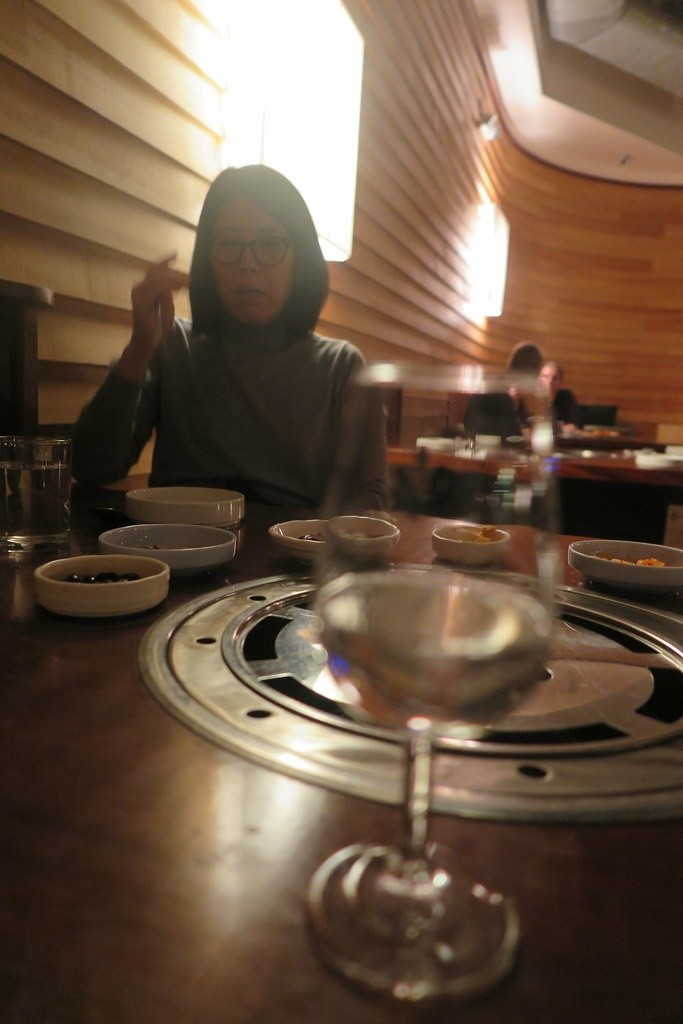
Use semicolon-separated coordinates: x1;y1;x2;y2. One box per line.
124;486;244;527
33;555;169;616
97;524;237;577
567;540;683;593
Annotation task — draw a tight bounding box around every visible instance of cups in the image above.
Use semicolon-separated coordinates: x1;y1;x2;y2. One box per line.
0;436;73;553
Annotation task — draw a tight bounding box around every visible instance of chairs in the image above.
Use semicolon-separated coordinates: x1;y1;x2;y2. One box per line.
341;382;404;513
579;404;620;437
443;394;497;436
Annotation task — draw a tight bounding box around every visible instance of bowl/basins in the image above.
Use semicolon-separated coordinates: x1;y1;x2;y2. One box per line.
432;525;510;564
325;515;399;559
268;519;329;566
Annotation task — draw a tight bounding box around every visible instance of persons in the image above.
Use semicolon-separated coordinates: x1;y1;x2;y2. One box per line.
508;361;583;431
65;164;389;517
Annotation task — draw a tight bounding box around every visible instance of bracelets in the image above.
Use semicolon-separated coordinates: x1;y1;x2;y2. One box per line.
109;359;151;386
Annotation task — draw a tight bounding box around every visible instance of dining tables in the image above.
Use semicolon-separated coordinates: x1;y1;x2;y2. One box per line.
387;445;683;545
0;505;683;1024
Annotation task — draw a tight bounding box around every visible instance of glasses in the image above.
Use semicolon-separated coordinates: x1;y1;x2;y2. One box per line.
207;234;294;266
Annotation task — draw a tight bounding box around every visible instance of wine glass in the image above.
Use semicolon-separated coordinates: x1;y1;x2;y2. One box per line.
305;362;566;1000
